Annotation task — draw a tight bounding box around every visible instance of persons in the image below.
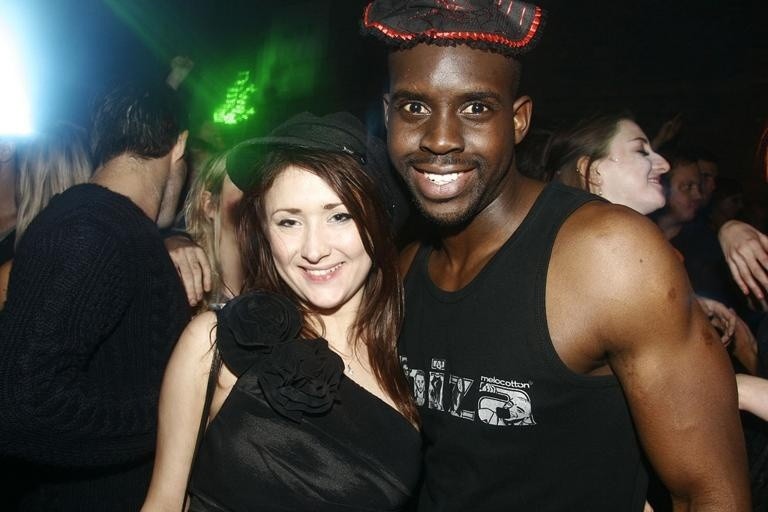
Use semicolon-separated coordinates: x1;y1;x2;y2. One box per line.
2;0;767;511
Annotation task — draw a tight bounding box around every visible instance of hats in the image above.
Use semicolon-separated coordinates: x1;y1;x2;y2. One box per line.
224;109;412;239
